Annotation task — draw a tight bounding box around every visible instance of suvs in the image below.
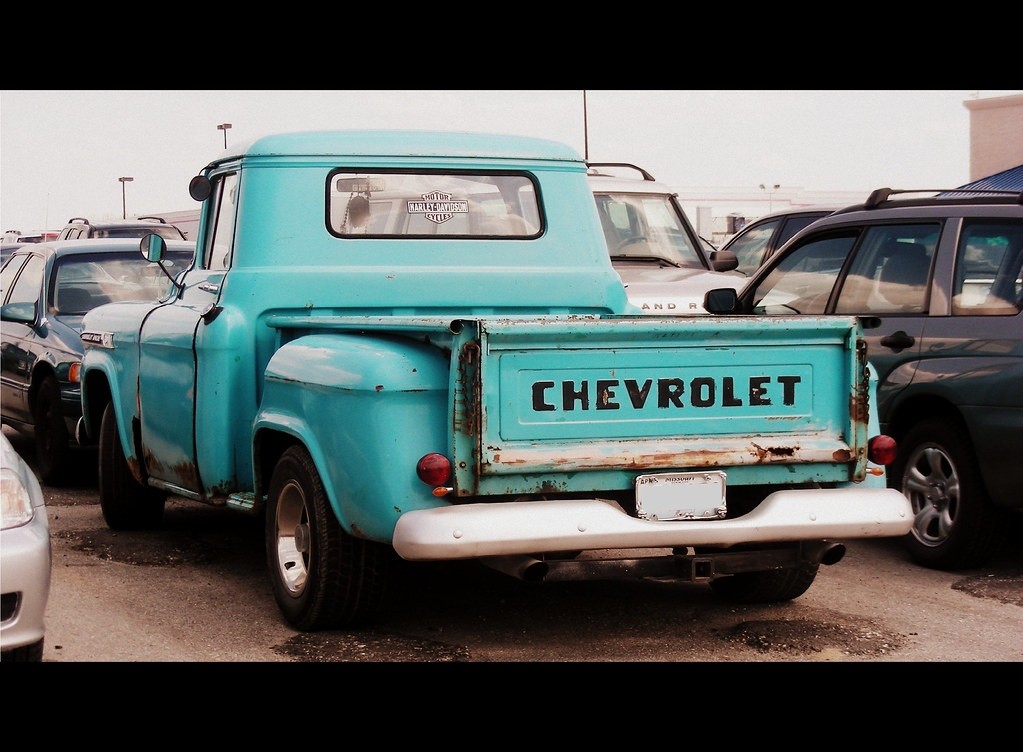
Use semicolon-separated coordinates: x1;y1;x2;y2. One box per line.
56;217;188;242
369;157;800;311
710;185;1023;573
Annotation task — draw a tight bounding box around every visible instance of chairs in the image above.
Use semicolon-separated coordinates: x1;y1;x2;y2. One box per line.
877;244;930;309
58;288;93;312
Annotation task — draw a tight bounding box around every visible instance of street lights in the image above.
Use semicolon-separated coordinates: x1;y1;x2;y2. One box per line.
119;177;133;220
759;184;780;214
217;124;232;150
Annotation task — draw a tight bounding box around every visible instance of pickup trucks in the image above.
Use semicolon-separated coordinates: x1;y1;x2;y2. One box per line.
72;133;916;633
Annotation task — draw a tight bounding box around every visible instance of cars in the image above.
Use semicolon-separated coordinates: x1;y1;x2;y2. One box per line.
0;240;206;487
0;429;52;661
